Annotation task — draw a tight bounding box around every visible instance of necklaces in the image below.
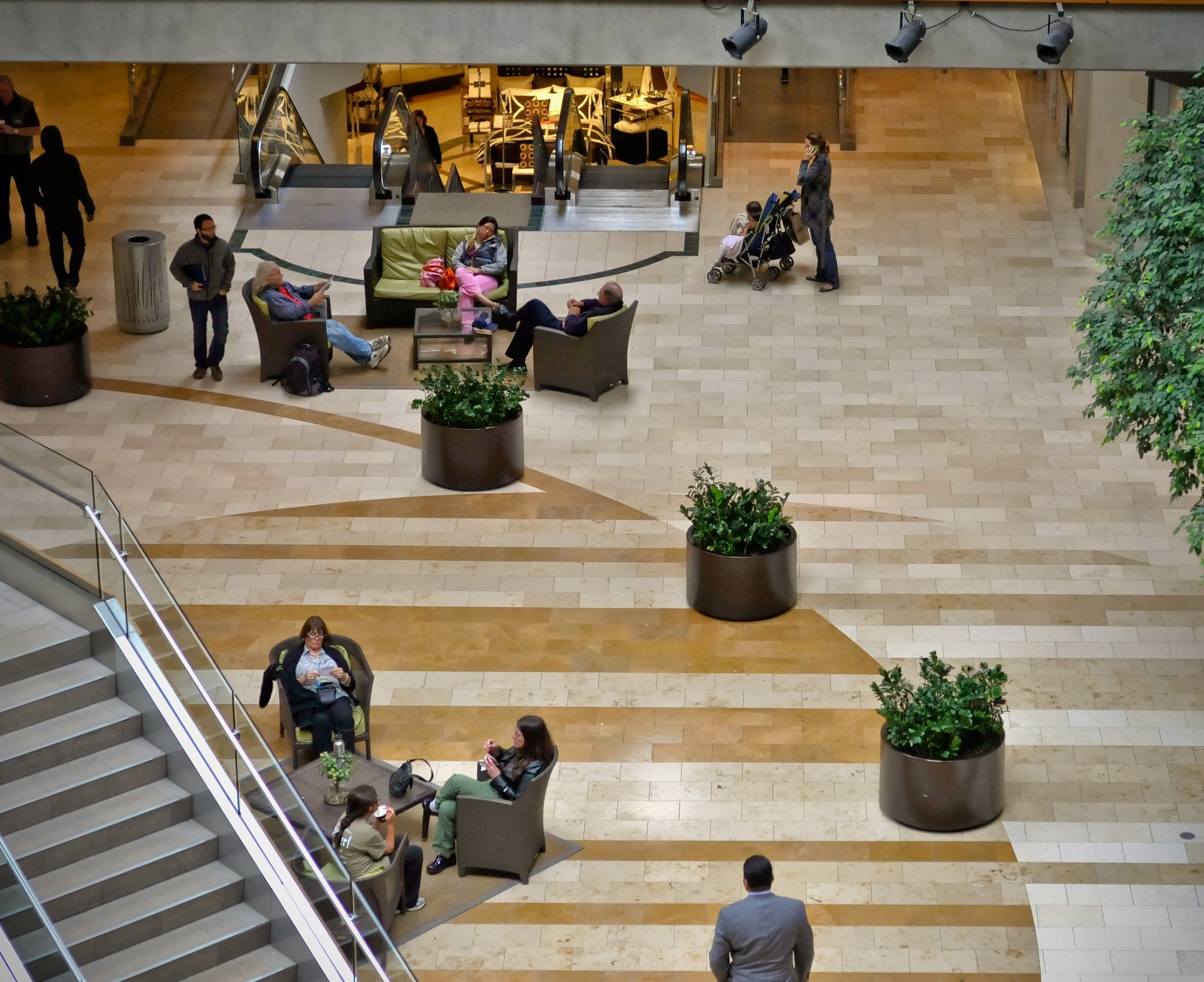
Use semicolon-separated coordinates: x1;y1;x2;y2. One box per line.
257;261;391;369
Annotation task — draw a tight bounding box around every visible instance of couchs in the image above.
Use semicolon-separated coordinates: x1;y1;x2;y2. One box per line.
362;224;520;333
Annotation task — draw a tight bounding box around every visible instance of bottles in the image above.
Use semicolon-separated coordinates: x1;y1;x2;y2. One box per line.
333;732;345;757
627;81;631;101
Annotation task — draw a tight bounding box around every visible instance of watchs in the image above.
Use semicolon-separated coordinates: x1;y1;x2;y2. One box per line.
14;128;18;135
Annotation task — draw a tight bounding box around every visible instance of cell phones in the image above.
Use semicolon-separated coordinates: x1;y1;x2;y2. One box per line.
808;145;819;152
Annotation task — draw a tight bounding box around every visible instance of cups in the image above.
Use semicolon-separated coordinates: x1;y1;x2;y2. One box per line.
637;98;646;104
373;806;389;823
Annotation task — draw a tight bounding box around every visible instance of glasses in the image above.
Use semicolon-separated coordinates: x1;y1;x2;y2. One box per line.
373;796;380;805
201;225;218;231
482;225;494;234
596;290;601;296
304;632;326;640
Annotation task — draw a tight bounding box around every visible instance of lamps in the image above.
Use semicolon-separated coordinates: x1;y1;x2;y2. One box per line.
721;0;769;61
1034;3;1076;66
884;0;928;64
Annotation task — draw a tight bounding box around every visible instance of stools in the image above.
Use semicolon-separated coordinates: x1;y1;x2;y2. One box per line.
611;128;668;165
511;164;538;192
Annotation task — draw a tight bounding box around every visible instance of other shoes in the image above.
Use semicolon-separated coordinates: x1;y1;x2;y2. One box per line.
27;236;38;247
819;285;838;293
806;276;827;283
0;233;12;245
212;365;223;381
192;366;208;378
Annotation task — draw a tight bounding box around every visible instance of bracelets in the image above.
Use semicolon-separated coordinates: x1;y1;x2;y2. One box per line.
340;673;349;684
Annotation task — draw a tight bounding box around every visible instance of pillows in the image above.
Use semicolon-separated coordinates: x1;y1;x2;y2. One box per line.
493;73;534;92
564;72;606;92
612;109;672;135
465;107;494;115
538;93;566;115
523;98;552;123
500;94;537;120
551;83;603;97
519;141;537;169
464;98;494;108
572;93;600;121
499;86;552;98
619;106;665;122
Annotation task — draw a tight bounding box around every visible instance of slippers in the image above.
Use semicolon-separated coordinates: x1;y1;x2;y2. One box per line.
491;301;499;315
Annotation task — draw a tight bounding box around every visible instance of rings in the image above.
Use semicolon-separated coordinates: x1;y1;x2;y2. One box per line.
337;674;339;676
311;677;313;679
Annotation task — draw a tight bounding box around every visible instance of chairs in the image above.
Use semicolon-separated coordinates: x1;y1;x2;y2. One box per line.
532;298;639;402
292;826;409;972
240;276;334;386
455;743;561;885
268;633;376;771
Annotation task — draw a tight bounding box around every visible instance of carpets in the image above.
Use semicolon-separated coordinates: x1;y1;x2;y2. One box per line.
231;743;585;970
325;312;544;392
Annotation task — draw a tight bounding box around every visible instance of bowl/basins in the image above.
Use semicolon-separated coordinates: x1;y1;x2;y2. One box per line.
648;90;665;98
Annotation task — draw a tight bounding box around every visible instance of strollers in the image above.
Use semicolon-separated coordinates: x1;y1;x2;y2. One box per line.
707;189;801;290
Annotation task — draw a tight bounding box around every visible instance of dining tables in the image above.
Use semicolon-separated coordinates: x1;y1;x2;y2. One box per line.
605;92;677;164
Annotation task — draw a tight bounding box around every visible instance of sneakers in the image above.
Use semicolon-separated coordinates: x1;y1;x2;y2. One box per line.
366;344;390;368
58;279;80;298
394;898;428;915
368;334;390;351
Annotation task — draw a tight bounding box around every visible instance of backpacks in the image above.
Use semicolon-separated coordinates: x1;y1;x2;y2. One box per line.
271;344;333;396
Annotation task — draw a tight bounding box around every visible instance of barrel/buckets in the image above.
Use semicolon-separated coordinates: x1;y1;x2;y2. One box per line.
631;84;641;98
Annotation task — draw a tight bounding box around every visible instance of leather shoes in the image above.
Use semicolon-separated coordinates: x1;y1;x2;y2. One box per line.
422;798;440;817
426;853;457;875
497;305;516;332
496;361;527;376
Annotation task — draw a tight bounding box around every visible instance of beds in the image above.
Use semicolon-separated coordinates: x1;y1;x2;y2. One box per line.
375;63;468;100
472;65;615;186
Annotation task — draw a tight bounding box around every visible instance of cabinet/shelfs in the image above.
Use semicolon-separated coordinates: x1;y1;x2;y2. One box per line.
345;62;387;133
460;86;493;154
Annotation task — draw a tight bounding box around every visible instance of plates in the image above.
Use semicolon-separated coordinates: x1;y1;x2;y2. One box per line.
633;102;648;106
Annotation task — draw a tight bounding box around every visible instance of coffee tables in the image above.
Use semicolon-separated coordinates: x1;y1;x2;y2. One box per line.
413;306;495;370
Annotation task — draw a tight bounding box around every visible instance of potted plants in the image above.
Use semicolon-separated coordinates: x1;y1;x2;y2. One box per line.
430;290;460;321
317;751;356;807
409;357;536;494
869;650;1011;835
0;275;103;408
676;461;801;622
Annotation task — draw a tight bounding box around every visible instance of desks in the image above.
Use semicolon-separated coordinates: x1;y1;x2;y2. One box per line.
244;750;437;862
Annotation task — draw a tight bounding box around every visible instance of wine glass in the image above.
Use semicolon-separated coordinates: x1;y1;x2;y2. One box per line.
612;81;617;99
619;81;623;98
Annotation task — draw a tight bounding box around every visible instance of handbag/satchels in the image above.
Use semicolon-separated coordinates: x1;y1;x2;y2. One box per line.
769;213;796;260
314;685;337;705
786;207;811;246
424;264;457;291
389;758;433;797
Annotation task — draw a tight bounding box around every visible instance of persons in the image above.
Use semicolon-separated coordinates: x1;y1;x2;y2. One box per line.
710;854;816;982
745;202;763;215
168;214;236;382
797;133;839;293
0;76;41;247
743;212;761;239
451;216;507;334
422;716;553;874
27;125;96;299
412;109;443;168
332;785;426;915
283;616;358;758
498;282;622;374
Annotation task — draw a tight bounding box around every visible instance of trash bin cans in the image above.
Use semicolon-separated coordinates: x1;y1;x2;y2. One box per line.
112;230;170;334
